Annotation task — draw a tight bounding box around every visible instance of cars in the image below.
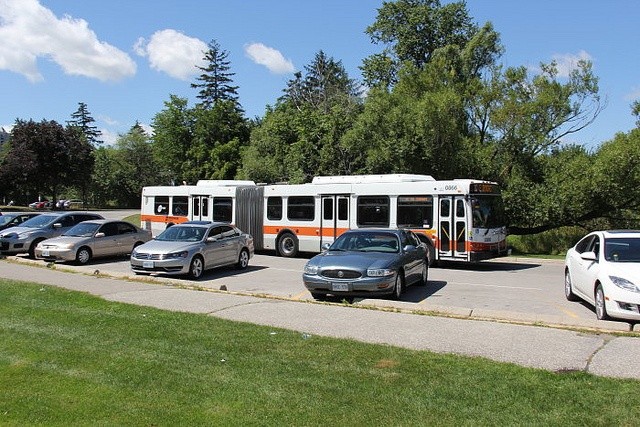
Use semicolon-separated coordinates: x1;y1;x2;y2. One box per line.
131;222;254;278
565;230;640;320
0;213;42;231
0;212;105;259
64;199;90;208
302;227;435;299
34;220;152;266
29;202;47;208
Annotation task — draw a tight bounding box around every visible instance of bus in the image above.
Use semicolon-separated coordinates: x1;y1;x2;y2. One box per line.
140;173;508;264
